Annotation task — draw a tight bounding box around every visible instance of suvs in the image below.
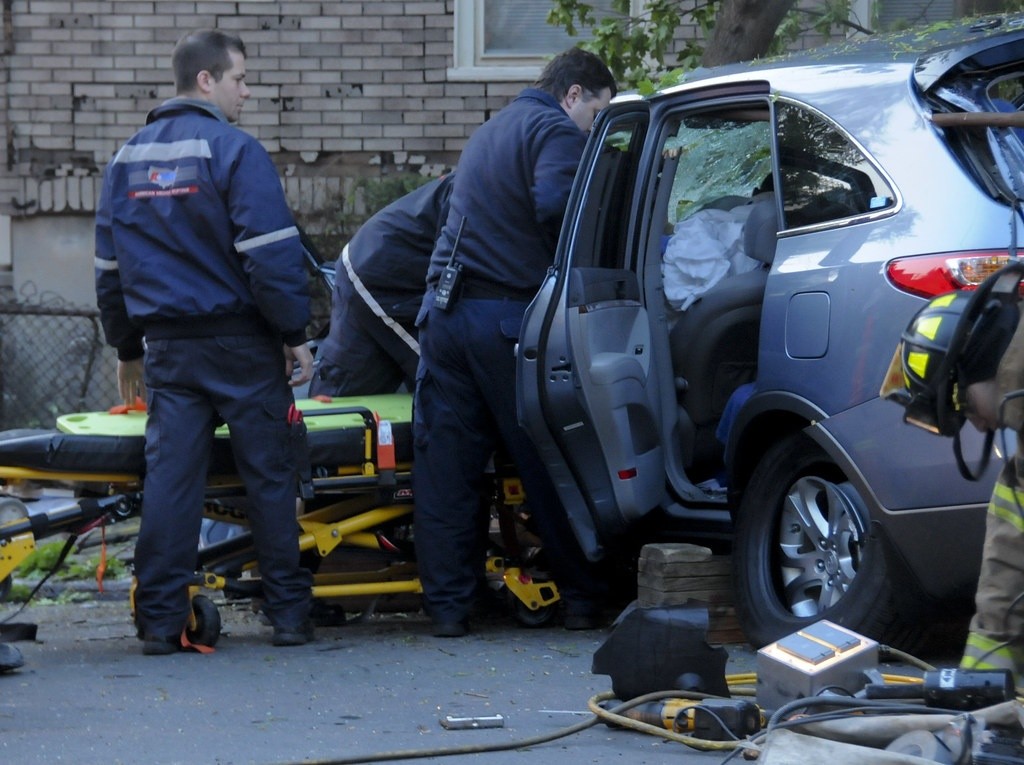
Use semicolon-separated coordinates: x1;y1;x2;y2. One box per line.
512;29;1022;669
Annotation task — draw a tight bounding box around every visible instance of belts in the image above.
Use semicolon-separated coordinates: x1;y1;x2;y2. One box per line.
454;282;511;301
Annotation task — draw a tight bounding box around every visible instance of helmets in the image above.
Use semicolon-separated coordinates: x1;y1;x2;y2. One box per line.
901;260;1024;435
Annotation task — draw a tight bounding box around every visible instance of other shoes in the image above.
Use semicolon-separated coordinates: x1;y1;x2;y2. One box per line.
144;631;180;652
273;607;313;643
565;614;600;629
433;617;468;636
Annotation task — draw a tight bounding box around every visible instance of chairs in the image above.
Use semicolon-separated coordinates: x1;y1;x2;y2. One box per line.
670;191;779;421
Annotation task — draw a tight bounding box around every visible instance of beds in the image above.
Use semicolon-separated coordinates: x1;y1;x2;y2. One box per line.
0;392;562;648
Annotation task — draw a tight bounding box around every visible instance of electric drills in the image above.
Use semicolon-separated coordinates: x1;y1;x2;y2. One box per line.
601;695;767;751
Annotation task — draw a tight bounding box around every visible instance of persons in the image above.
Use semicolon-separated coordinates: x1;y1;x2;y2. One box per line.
93;30;318;655
961;303;1023;691
308;170;458;397
410;44;618;637
